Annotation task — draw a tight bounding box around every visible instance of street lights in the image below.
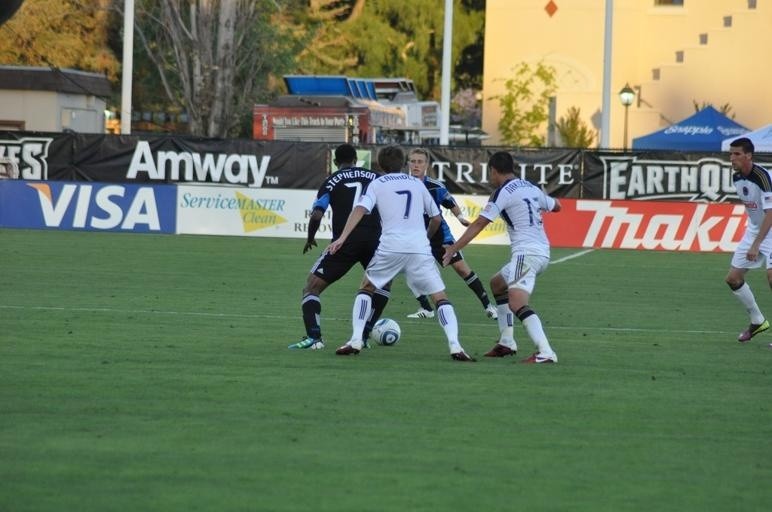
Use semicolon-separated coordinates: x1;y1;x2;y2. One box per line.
615;83;635;150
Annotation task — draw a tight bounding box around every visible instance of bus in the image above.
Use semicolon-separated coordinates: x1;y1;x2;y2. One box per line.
104;97;190;135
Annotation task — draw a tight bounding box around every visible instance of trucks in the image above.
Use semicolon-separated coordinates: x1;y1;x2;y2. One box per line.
251;72;489;147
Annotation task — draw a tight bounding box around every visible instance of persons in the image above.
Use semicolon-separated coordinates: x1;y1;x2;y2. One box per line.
404;147;499;320
326;144;474;360
441;151;563;364
724;136;772;350
287;142;387;352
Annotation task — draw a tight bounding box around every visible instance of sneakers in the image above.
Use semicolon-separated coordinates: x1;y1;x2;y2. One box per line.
484;303;498;321
483;341;517;358
334;340;361;356
362;339;371;349
737;317;770;342
286;335;326;351
406;307;437;318
512;349;558;366
449;347;477;362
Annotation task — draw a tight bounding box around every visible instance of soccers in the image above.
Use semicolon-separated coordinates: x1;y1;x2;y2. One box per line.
371;319;400;346
